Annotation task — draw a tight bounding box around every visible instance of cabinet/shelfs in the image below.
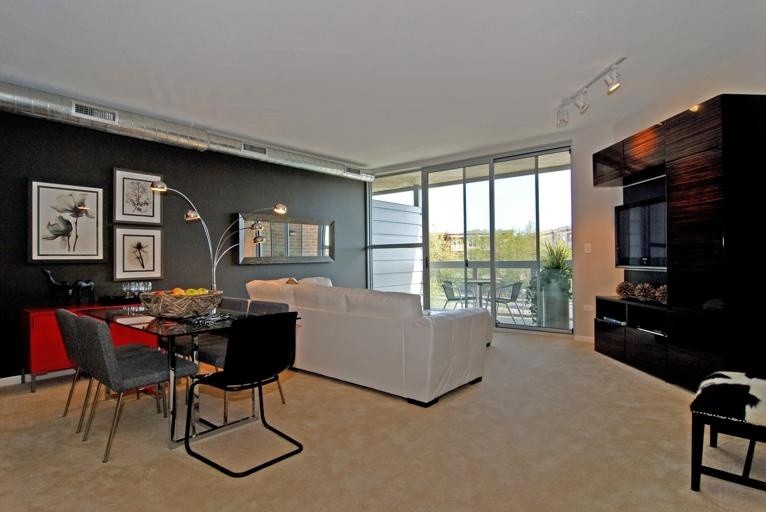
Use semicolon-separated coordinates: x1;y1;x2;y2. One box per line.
592;94;766;397
16;295;160;394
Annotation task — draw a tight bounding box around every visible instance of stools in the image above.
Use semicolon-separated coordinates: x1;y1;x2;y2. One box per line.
686;370;766;494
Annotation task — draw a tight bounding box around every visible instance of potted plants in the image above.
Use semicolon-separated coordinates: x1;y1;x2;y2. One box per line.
541;237;571;277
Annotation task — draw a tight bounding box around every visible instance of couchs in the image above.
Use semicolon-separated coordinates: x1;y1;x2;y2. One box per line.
243;274;495;408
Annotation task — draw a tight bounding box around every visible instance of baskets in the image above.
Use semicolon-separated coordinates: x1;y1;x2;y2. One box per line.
139;288;224;320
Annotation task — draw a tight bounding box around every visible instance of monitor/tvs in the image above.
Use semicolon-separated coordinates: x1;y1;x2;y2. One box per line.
615;204;673;272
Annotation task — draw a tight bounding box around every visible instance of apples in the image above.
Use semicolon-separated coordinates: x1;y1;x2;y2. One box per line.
154;287;209;296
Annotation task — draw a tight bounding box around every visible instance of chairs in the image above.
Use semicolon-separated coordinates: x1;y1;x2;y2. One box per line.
188;302;287;429
183;299;246;406
484;281;528;326
55;310;161;431
440;279;477;310
184;311;303;476
76;317;197;463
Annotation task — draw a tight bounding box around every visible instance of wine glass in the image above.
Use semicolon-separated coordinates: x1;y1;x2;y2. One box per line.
122;282;153;299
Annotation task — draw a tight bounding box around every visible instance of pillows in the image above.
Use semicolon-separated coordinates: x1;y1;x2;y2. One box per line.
297;276;332;288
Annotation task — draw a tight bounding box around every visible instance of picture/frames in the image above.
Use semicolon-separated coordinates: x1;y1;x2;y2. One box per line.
26;178;109;265
110;224;166;282
110;166;164;227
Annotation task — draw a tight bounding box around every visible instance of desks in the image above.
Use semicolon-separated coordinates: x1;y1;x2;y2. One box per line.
460;279;504;307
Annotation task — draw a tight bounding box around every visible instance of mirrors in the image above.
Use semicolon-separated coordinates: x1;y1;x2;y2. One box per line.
237;212;338;266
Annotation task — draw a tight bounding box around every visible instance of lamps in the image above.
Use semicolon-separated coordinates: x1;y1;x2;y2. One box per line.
556;60;621;127
149;181;287;297
